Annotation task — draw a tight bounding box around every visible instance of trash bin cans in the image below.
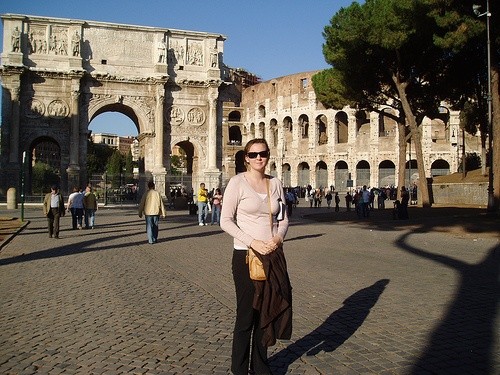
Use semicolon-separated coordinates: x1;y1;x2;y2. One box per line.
7;187;18;209
188;204;197;215
173;197;187;209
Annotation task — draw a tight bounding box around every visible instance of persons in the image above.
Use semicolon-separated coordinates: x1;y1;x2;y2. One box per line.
197;183;223;227
43;185;65;239
168;185;194;209
352;182;418;220
71;29;80;56
325;191;332;209
137;181;166;244
157;37;219;67
25;32;68;55
283;184;327;218
67;185;97;230
344;191;352;211
219;138;289;375
334;192;340;212
11;25;21;53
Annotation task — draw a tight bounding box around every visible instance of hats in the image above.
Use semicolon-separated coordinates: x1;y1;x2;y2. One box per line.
52;184;57;189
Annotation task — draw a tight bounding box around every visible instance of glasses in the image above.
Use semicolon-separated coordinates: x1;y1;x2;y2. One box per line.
246;150;269;159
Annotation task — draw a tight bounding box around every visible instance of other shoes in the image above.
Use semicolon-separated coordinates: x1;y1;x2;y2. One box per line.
211;223;213;225
205;223;208;225
55;236;58;238
49;235;52;238
78;223;82;230
85;226;89;229
199;223;204;226
91;226;95;229
217;223;220;226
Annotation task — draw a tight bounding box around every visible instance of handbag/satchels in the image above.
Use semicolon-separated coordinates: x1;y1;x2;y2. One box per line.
248;249;266;280
